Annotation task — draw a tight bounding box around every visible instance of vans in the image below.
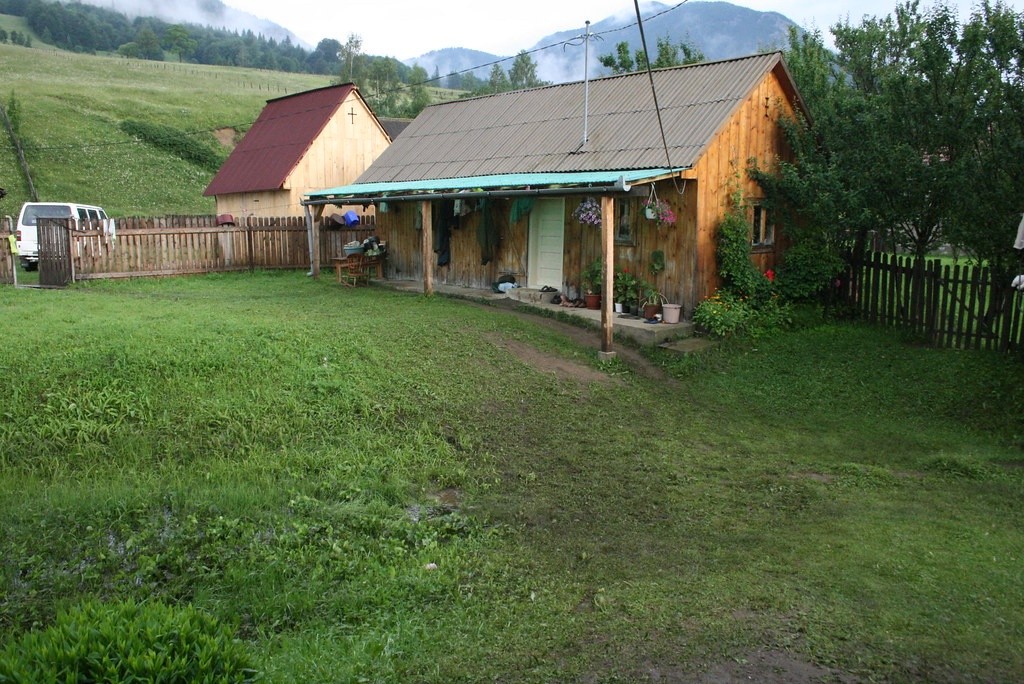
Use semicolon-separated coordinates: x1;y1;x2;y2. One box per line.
16;202;109;273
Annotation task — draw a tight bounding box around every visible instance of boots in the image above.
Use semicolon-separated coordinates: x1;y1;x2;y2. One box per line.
557;299;561;303
559;294;567;305
564;302;575;307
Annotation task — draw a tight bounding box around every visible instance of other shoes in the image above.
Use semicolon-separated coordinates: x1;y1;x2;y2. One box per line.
575;301;581;307
579;302;586;307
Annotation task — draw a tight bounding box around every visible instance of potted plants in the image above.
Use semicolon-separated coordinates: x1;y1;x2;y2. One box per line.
613;273;661;320
581;257;602;310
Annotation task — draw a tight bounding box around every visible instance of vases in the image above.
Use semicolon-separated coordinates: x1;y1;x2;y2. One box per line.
645;208;656;219
662;304;682;323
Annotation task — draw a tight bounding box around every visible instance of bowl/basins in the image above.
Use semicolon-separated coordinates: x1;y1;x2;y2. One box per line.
344;247;364;255
216;214;235;226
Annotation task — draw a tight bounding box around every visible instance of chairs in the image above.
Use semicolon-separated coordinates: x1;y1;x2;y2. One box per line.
346;252;369;286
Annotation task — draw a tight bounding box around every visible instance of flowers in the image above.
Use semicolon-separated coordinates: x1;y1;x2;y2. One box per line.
572;196;601;224
640;199;678;230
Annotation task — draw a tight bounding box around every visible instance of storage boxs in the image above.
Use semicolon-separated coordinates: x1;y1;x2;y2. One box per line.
344;210;359;228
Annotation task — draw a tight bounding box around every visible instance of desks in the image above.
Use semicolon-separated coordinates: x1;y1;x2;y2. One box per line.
331;255;382;283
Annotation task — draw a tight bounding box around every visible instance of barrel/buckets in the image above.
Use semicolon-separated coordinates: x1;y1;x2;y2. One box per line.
343;211;360;227
663;304;682;323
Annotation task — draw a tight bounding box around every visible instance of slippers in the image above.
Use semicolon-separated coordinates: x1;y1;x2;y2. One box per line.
540;285;557;292
554;299;559;304
